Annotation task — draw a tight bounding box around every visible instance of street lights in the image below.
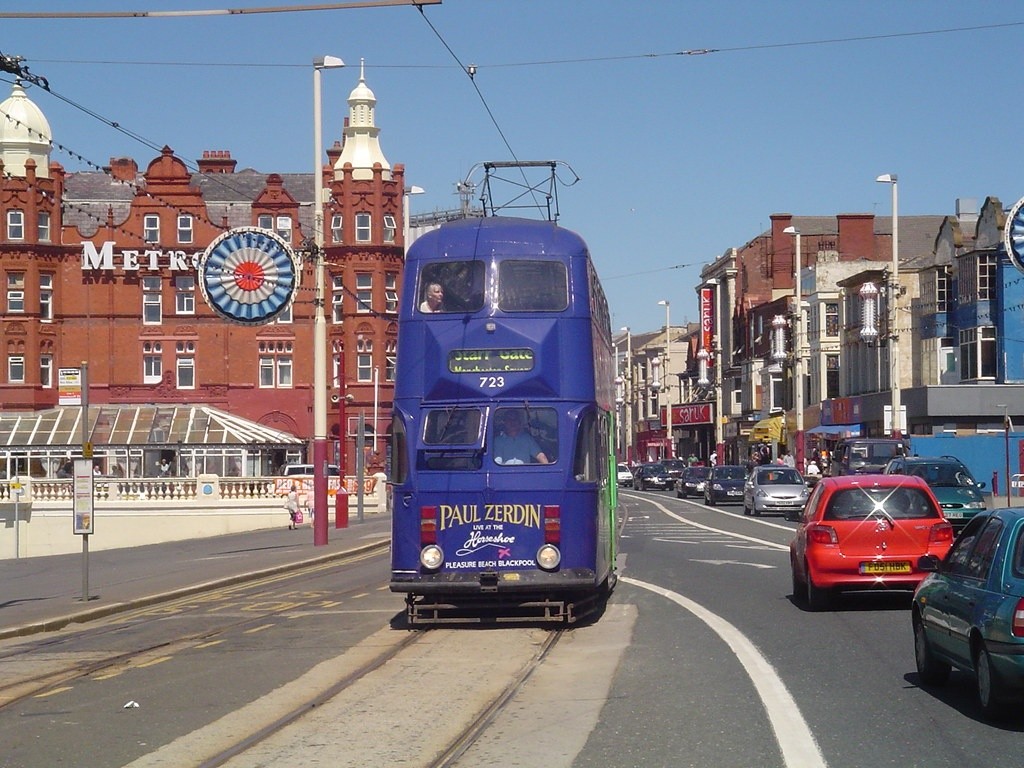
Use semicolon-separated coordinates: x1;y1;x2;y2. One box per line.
876;172;903;440
622;327;632;467
612;342;620;464
401;184;426;262
658;300;672;459
308;51;346;544
706;278;723;465
781;224;806;474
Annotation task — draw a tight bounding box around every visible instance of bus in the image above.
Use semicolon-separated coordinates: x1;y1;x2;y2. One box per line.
386;157;618;627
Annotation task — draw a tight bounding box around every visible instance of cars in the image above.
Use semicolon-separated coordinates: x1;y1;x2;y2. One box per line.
742;464;809;517
676;466;713;499
617;458;687;491
910;503;1024;722
783;473;955;611
703;465;750;506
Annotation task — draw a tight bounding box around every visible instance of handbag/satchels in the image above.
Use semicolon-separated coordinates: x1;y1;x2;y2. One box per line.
295;510;303;523
284;494;289;509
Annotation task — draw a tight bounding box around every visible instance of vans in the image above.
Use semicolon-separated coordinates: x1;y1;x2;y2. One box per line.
282;463;340;476
831;436;912;478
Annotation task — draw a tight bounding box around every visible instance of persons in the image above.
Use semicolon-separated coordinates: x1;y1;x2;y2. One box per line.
112;466;123;478
307;486;315;529
58;462;73;477
93;466;101;475
688;454;698;462
709;450;717;467
807;461;820;474
760;445;770;464
811;452;820;465
494;410;549;463
161;456;175;475
752;452;759;462
783;450;795;467
819;449;828;460
280;460;287;474
420;283;445;312
287;486;301;529
777;454;784;465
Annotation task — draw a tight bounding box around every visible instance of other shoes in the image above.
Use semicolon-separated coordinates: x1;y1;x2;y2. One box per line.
289;526;291;530
292;528;297;530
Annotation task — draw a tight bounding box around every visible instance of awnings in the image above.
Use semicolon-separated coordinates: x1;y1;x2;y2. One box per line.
748;416;787;446
806;423;866;440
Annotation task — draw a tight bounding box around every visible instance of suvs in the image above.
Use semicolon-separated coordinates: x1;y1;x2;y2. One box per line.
879;455;987;536
